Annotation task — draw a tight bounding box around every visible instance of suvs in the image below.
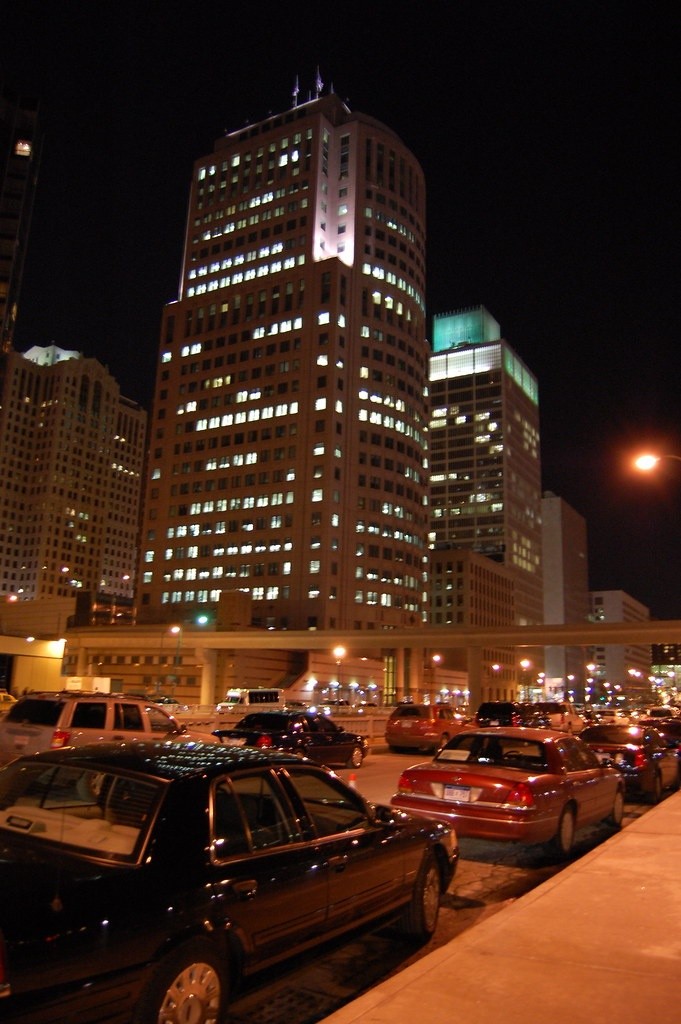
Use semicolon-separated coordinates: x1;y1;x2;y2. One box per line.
0;688;245;781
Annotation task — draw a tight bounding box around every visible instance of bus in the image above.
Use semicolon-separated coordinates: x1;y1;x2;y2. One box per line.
215;688;287;716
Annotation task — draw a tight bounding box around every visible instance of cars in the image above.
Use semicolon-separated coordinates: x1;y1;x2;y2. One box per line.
318;699;349;714
575;725;681;804
210;711;370;768
390;726;627;858
0;739;461;1024
384;705;477;752
475;702;681;762
285;701;309;711
351;700;378;712
150;696;179;704
0;690;20;713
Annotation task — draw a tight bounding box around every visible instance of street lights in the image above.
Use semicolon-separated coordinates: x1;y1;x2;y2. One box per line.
429;655;442;719
519;659;531;701
168;616;209;703
492;665;499;704
335;646;345;712
155;625;181;694
